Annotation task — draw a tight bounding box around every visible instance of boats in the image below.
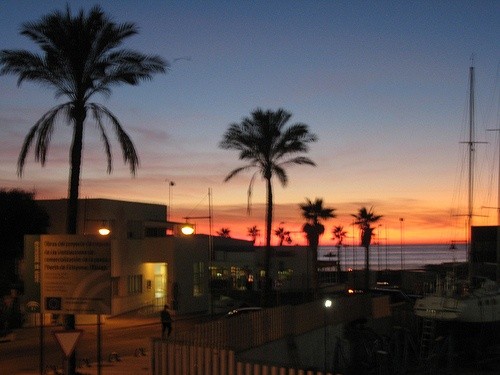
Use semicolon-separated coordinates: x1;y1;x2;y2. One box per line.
415;278;499;322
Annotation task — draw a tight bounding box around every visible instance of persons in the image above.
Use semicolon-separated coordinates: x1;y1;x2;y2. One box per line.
160;304;172;339
